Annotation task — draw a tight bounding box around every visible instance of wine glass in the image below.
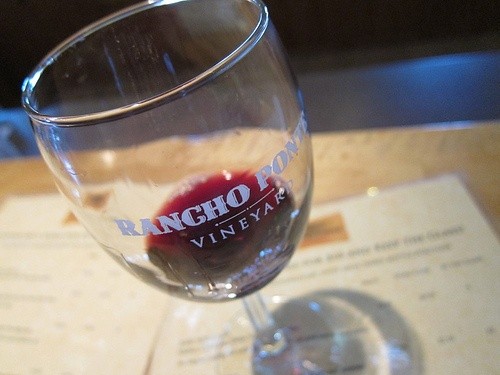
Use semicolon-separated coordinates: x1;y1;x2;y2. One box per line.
20;0;391;375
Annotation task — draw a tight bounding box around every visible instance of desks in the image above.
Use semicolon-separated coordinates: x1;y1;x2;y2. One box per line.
2;116;500;375
